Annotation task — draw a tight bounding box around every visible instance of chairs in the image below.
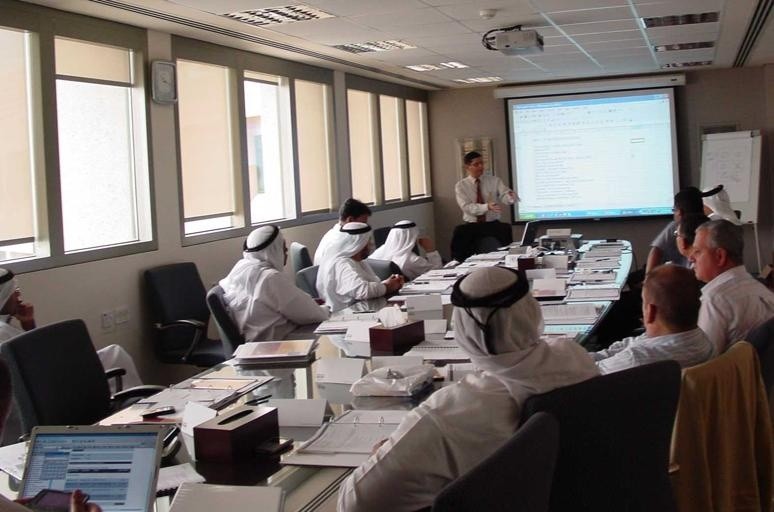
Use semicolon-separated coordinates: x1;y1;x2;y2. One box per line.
136;262;229;382
0;318;164;447
517;358;685;511
749;315;774;395
351;413;561;512
667;341;768;510
208;222;510;362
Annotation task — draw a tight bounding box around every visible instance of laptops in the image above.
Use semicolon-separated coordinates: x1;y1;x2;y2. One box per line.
19;422;164;512
500;220;540;249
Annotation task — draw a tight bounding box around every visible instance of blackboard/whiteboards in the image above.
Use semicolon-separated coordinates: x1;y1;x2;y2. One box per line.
699;129;764;224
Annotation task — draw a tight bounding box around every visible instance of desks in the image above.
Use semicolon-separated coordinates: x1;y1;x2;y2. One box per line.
0;240;633;511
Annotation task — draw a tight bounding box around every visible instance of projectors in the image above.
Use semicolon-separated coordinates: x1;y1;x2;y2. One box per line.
495;29;543;56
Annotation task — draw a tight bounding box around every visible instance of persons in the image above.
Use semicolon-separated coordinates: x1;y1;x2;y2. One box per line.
0;360;107;512
580;185;772;378
0;270;142;415
218;197;446;336
455;152;520;223
337;265;599;512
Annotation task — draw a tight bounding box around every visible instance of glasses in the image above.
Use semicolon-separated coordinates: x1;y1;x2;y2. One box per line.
673;231;684;240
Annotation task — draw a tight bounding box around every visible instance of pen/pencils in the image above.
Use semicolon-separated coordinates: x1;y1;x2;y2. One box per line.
444;275;455;277
353;310;375;314
245;395;272;404
255;400;268;405
596;258;610;261
414;282;429;284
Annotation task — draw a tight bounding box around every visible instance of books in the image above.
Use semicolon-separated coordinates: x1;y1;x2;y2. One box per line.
464;242;623;325
140;268;470;468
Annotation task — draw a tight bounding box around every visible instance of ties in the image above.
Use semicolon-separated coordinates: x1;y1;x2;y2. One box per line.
476;178;486;223
